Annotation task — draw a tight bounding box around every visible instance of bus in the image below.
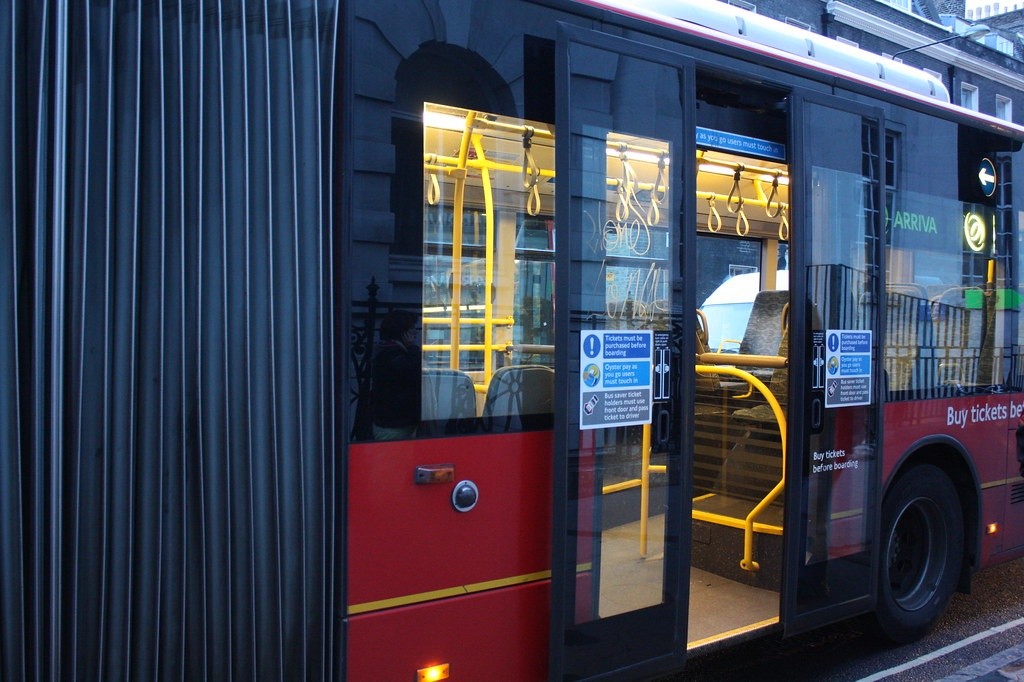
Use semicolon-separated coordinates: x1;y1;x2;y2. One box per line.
2;0;1023;682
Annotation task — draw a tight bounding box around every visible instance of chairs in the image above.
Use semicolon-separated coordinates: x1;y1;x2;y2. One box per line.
482;365;556;417
422;368;476;421
605;283;1024;430
